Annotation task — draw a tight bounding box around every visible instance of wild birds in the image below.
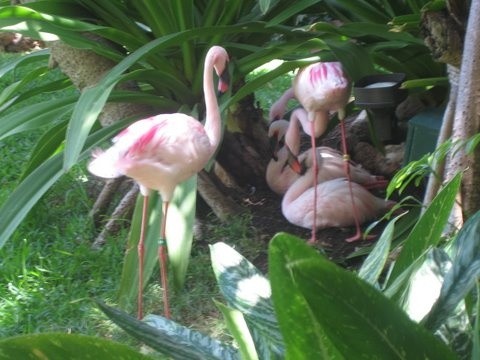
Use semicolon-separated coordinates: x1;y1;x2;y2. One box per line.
87;45;231;321
266;60;378;247
268;108;389;195
281;144;406;232
